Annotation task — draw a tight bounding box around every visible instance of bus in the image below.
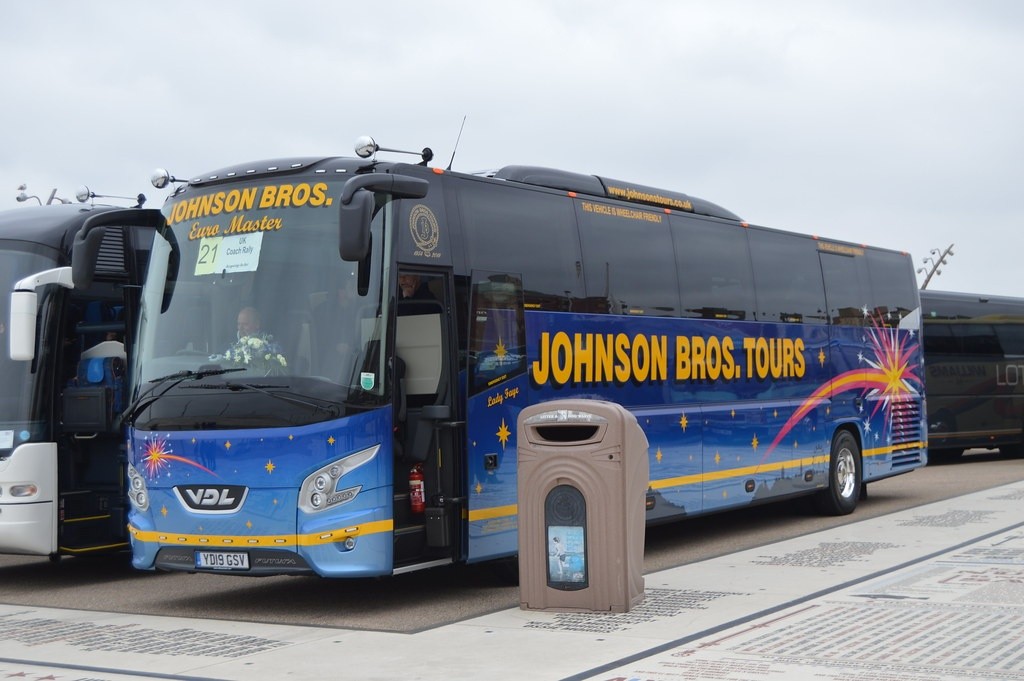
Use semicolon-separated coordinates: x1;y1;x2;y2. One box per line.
919;290;1024;462
121;116;929;581
0;183;163;571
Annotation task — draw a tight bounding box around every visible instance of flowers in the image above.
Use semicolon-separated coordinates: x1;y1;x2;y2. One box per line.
220;328;289;369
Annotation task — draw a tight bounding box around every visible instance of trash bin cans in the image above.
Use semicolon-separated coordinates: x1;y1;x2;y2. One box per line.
516;398;651;613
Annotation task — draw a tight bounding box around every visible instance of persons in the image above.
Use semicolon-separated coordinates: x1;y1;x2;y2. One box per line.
399;275;437;300
220;306;293;369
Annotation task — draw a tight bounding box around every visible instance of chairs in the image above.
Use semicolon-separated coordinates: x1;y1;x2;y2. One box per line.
353;335;405;459
277;308;320;375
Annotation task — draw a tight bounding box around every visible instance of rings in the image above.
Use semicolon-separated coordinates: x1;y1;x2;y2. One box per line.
412;285;415;287
